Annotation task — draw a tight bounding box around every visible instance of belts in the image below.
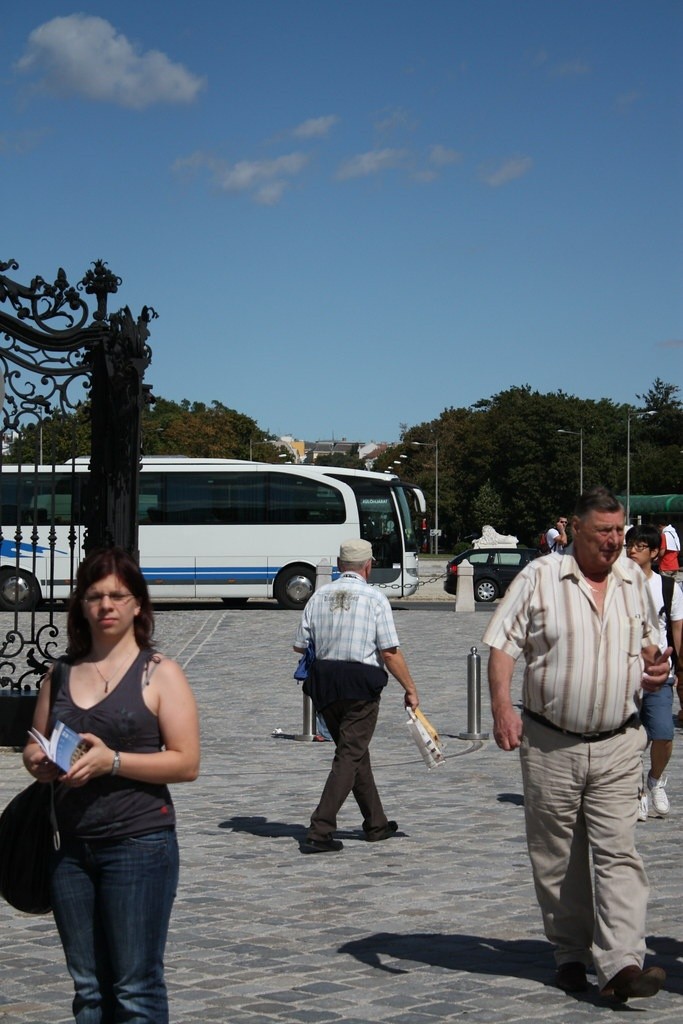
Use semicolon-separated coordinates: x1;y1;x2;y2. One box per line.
523;707;636;743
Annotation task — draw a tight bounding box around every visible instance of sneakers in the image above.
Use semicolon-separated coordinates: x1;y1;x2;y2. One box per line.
647;771;670;814
637;793;648;821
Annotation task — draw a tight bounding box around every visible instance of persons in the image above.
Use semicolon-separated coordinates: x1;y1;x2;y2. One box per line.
544;514;567;554
293;540;419;852
651;512;680;577
481;486;673;1003
22;550;200;1024
624;525;683;821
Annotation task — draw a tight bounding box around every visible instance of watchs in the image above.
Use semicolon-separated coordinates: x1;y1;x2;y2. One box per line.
110;750;120;775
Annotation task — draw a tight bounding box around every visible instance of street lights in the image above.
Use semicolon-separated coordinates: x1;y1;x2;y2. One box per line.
557;429;583;496
411;441;439;556
384;455;433;554
249;439;276;461
627;411;658;524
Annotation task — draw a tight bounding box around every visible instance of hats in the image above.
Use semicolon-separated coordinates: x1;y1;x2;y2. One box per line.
339;539;376;562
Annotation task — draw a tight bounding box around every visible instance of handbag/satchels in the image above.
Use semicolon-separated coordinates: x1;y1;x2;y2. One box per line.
0;777;57;914
536;543;551;557
406;706;446;770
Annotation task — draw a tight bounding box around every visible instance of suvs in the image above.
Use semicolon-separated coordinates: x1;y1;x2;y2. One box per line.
443;547;545;603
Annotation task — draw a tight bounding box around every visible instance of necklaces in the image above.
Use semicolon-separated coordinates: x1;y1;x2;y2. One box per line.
90;645;138;693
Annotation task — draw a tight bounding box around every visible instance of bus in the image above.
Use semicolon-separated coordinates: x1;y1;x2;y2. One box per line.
0;458;426;611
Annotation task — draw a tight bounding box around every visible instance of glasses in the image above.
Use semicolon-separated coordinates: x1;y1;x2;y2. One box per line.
84;588;136;607
561;521;568;524
624;541;649;553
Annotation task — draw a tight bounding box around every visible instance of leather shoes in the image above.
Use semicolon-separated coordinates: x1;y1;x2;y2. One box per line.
366;821;398;842
600;966;666;1005
554;963;588;992
300;838;344;854
